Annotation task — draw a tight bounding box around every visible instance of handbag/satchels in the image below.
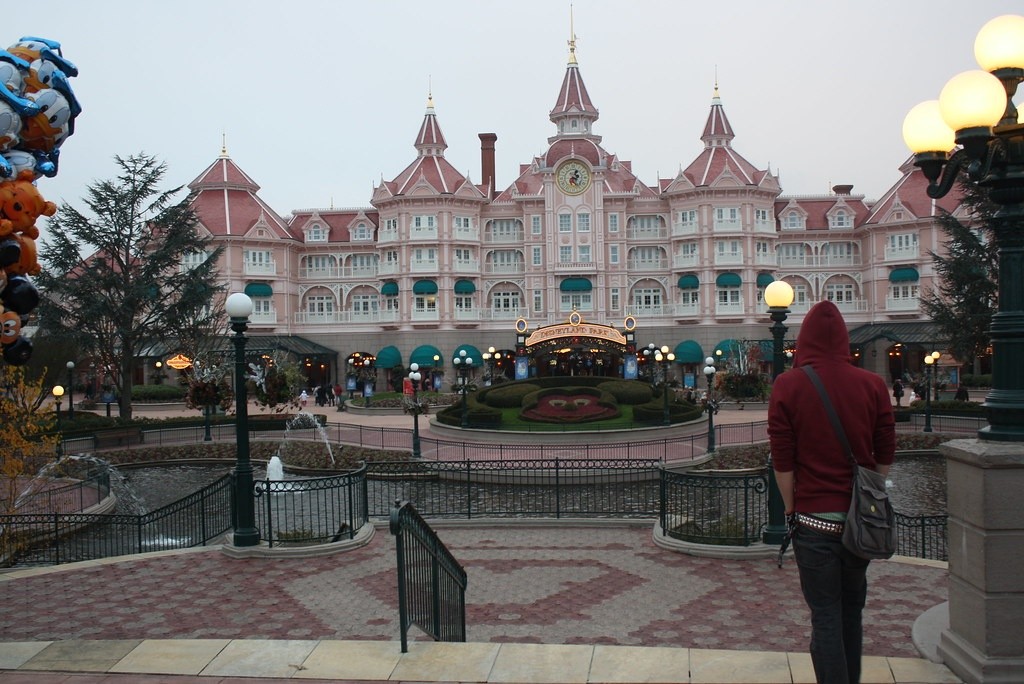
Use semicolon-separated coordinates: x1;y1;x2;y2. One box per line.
841;466;898;559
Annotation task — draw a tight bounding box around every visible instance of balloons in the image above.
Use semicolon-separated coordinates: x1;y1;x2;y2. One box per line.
0;37;82;365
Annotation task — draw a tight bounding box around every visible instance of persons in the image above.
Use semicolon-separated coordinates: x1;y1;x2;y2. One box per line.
954;381;969;402
909;380;925;403
681;386;697;405
299;391;310;406
701;390;707;412
768;301;896;684
893;379;904;406
422;378;430;391
313;384;342;407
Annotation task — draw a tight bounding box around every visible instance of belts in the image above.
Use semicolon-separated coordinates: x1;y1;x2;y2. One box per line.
795;513;843;534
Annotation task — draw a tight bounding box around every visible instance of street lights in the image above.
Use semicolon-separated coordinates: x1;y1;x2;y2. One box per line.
224;292;261;548
765;280;795;378
409;363;422;458
348;359;354;399
642;343;661;378
703;356;717;453
53;385;64;462
716;349;722;363
363;359;371;407
65;361;74;423
103;363;112;416
655;345;675;426
902;13;1024;445
433;355;440;393
931;352;941;401
482;346;502;387
924;355;935;433
453;349;474;430
155;361;163;385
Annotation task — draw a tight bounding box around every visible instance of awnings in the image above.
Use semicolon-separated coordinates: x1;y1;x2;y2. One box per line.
713;339;744;361
757;273;774;286
716;273;742;286
760;342;774;361
678;275;699;288
674;340;703;364
380;283;398;294
453;343;484;368
245;283;273;296
560;278;591;291
889;268;919;282
410;345;443;367
413;281;438;293
374;346;402;368
